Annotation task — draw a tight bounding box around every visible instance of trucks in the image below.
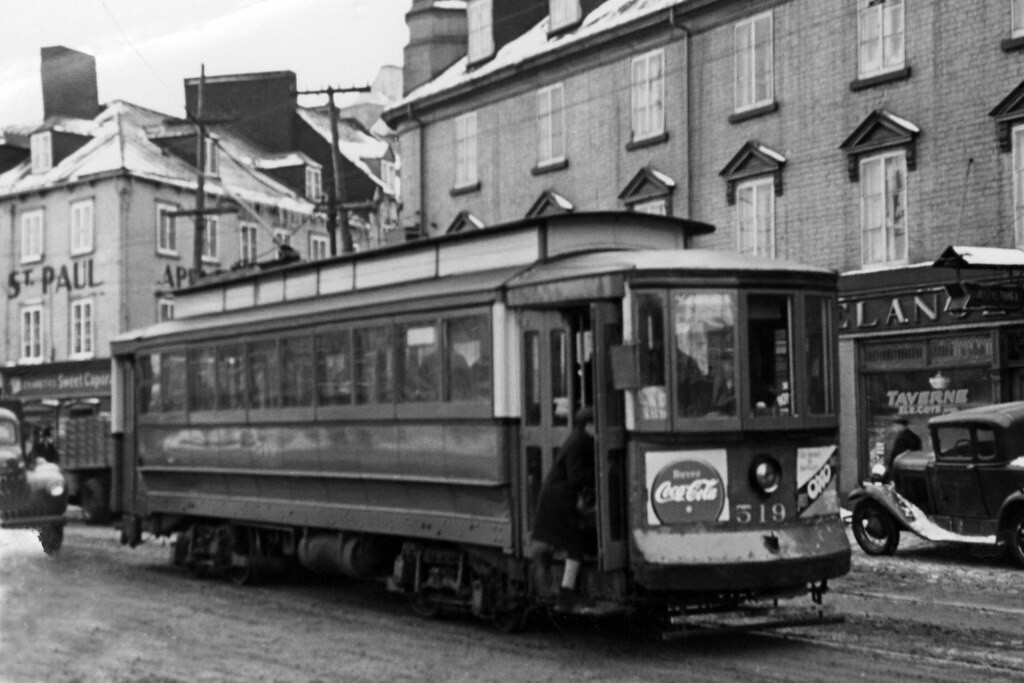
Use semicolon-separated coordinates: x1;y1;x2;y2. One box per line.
0;409;92;554
22;397;111;523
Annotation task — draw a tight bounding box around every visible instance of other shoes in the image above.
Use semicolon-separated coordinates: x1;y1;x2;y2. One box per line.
553;586;589;614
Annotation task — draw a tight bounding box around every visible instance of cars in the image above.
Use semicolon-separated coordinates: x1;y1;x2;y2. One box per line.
844;398;1023;568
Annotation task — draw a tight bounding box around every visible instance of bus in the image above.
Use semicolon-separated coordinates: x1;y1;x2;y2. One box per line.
104;202;852;638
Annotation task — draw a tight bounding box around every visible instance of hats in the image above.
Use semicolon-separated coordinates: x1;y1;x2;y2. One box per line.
890;415;908;425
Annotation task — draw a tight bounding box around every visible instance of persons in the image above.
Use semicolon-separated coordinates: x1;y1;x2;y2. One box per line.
532;408;596;608
888;416;922;469
